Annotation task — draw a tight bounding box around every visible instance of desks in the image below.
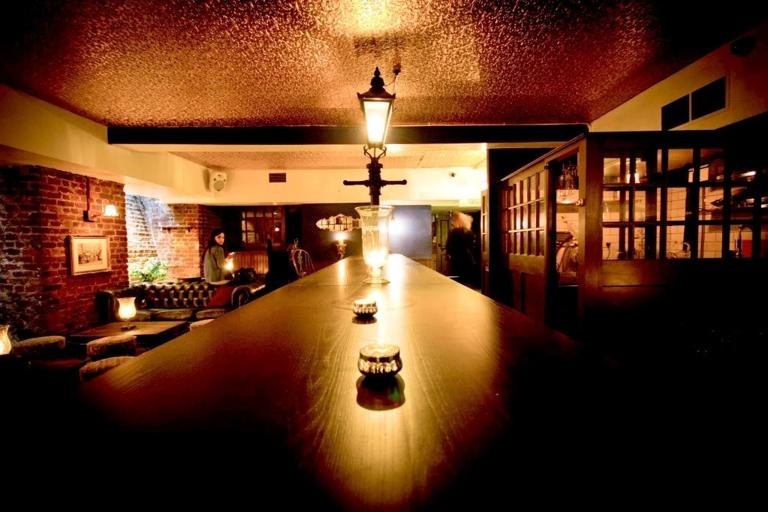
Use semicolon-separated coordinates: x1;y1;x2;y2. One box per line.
84;248;575;511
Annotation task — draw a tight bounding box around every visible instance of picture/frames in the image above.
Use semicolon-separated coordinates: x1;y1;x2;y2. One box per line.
68;233;113;278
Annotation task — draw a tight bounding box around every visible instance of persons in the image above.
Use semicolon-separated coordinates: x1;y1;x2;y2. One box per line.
445;211;477;273
203;228;233;283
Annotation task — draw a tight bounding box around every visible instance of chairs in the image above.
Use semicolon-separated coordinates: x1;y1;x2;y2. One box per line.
292;249;314;279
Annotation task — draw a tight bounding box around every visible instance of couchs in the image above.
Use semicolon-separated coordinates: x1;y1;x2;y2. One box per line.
98;280;267;325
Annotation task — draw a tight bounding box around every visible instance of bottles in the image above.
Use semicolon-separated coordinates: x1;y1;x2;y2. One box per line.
351;312;379;325
354;372;406;411
558;161;578;204
352;298;379;317
357;343;402;375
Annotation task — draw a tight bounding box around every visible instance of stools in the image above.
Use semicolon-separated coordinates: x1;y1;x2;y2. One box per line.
11;318;220;382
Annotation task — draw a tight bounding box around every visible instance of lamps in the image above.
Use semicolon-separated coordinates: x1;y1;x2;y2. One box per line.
115;296;137;330
343;64;407;206
86;204;118;223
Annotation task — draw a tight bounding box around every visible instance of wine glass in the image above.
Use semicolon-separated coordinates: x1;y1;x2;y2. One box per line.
355;205;398;285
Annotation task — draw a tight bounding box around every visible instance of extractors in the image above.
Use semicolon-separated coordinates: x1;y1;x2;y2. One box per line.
687;161;757;182
